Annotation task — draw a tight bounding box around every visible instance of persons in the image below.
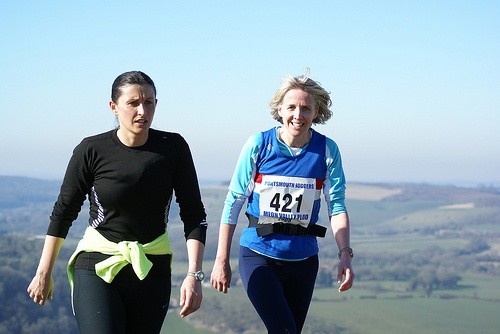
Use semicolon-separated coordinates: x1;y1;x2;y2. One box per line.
210;76;356;334
27;70;207;334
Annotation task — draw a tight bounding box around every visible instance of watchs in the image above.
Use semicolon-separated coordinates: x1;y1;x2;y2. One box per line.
338;248;353;260
186;270;204;281
